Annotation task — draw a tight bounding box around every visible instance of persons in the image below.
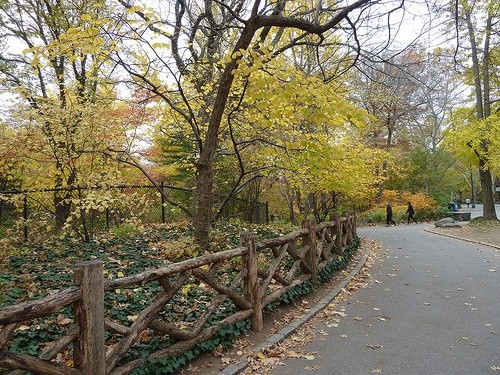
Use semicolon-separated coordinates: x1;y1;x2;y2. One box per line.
465;197;471;208
405;201;420;225
385;201;396;228
